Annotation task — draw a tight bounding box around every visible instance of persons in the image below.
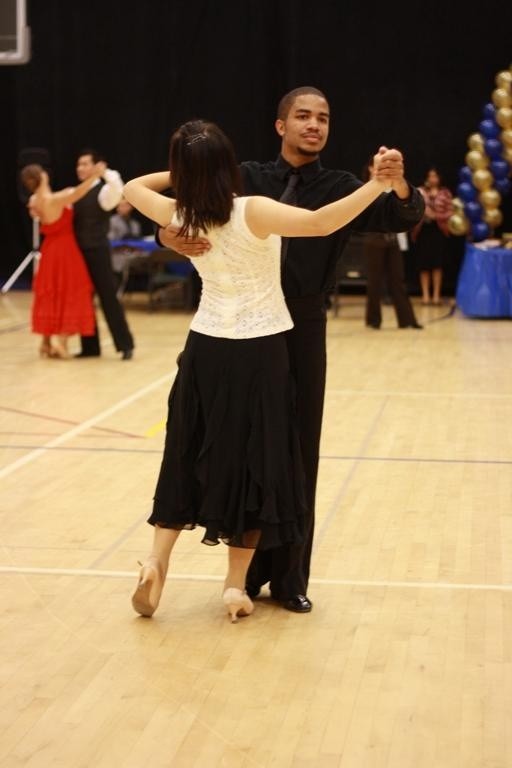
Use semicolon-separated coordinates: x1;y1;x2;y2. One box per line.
120;119;400;622
20;160;112;359
362;152;424;331
72;147;136;363
410;165;454;307
106;199;158;272
156;86;423;611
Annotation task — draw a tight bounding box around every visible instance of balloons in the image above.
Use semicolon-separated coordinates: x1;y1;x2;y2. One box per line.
447;63;512;252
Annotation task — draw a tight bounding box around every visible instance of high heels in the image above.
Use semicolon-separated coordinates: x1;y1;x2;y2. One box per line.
50;341;74;359
39;343;51;357
132;557;161;618
222;587;255;624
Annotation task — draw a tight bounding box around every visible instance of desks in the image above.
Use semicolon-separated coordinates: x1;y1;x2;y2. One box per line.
457;247;512;318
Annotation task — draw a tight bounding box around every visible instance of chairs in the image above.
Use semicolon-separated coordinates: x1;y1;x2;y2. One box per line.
334;238;369;317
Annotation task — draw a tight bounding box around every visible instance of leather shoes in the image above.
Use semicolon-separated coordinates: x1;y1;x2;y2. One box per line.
123;350;132;360
401;322;423;329
271;589;312;612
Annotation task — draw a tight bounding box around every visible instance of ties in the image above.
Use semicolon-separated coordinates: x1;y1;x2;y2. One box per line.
278;174;302;271
